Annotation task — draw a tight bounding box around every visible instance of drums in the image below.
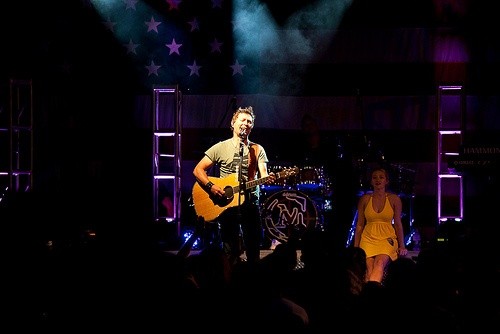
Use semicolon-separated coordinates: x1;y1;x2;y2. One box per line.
258;189;320;242
265;163;288;187
291;166;327;190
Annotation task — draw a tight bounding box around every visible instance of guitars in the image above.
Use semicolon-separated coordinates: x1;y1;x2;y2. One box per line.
193;165;302;224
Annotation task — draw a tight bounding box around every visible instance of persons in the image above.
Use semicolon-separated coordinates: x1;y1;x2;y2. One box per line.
193;107;276;279
354;169;408;283
1;184;500;334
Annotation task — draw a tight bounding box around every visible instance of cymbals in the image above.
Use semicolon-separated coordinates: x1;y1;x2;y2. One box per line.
393;163;415;175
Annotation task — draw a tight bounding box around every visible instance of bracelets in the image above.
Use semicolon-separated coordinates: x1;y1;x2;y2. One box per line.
205;181;214;191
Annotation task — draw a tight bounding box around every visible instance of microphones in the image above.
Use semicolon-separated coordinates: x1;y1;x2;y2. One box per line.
241;129;248;136
